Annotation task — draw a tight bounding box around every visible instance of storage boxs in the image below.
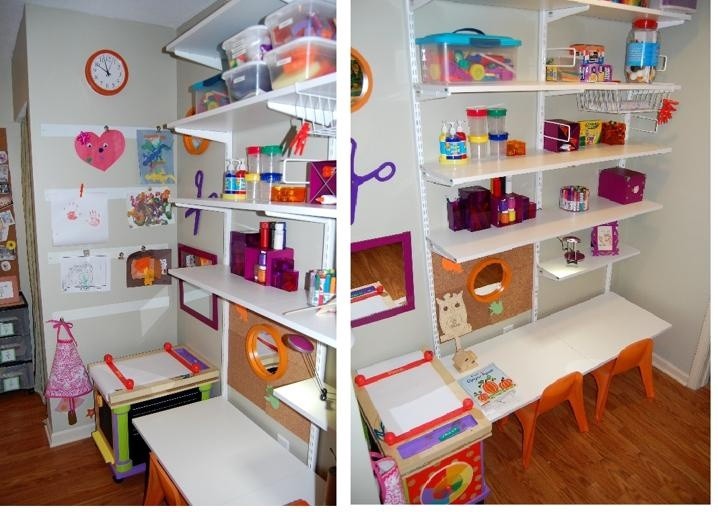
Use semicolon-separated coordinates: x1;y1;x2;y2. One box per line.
188;1;336;116
545;55;584;83
415;25;523;85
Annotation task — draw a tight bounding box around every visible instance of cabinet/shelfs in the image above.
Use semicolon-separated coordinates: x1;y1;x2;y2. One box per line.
406;0;692;283
163;1;353;434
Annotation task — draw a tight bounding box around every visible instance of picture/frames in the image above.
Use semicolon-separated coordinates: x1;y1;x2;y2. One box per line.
591;221;620;257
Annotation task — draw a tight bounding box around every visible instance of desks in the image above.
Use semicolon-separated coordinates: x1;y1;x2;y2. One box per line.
439;290;672;426
131;398;330;506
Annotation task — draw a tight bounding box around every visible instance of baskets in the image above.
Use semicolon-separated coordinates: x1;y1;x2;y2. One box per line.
575;88;674;113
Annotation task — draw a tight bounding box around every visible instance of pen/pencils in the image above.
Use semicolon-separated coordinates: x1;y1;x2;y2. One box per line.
560;185;590;212
305;268;335;309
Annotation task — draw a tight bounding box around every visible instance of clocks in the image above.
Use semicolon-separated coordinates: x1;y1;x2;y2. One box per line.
85;48;128;97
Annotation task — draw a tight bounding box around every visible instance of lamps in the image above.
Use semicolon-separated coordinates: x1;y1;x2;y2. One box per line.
283;334;335;403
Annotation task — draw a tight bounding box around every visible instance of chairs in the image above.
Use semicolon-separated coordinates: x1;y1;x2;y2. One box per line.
143;451;186;506
498;337;654;470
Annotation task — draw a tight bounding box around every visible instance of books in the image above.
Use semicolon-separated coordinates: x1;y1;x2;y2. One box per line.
458;361;522;413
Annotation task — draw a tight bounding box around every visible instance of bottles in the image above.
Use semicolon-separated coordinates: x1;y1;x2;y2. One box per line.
622;16;661;84
245;144;284;205
464;105;509;164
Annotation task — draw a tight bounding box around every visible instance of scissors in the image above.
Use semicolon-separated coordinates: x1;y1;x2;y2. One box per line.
185;171;218;235
350;138;396;226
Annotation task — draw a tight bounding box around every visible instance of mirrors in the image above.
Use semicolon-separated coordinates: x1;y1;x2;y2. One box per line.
174;243;219;330
350;49;373;112
469;260;513;304
350;230;416;330
244;326;284;381
